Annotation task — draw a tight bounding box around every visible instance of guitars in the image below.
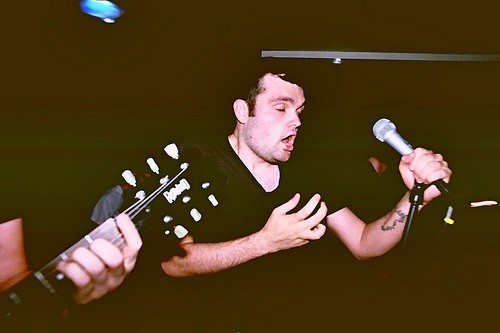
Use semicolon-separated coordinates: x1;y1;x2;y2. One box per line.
0;141;217;333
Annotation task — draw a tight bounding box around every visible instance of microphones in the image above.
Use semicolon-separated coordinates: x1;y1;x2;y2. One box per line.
372;118;452;197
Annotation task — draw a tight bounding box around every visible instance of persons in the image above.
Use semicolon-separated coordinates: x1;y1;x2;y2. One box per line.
150;69;452;333
0;148;143;333
